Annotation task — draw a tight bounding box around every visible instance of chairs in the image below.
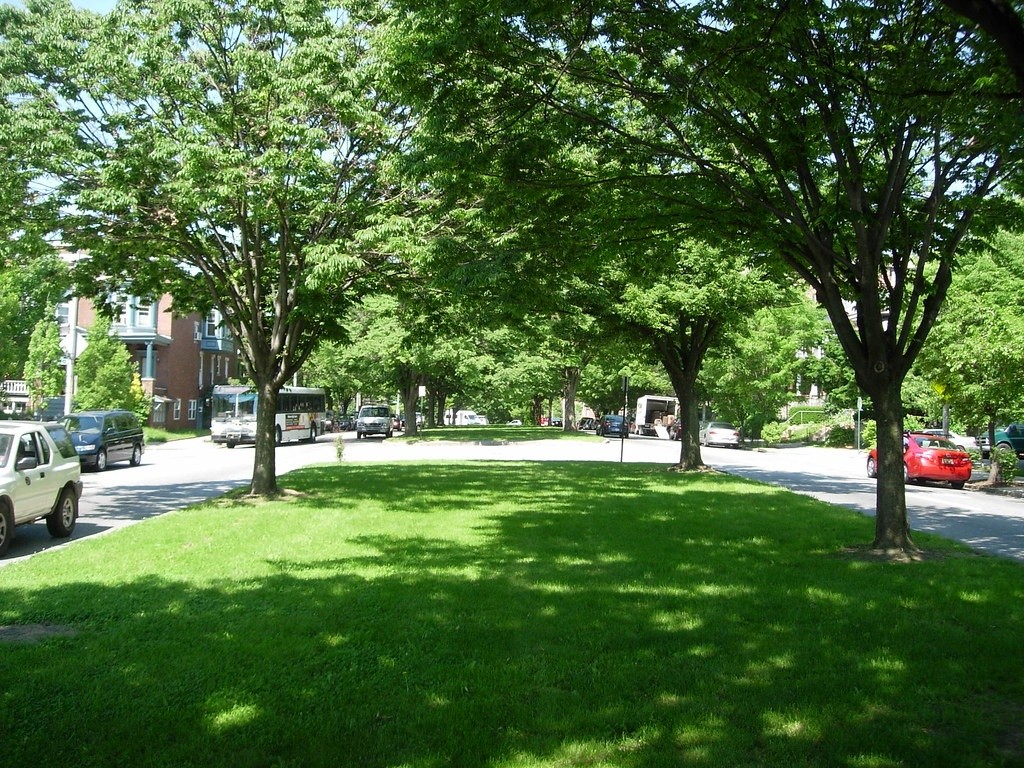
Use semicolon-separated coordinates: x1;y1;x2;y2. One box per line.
929;442;938;448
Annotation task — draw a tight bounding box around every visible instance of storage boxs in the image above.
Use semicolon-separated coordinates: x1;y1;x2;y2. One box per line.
653;411;675;424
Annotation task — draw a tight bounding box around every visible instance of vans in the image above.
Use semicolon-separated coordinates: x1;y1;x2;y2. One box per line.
442;408;489;426
355;403;395;439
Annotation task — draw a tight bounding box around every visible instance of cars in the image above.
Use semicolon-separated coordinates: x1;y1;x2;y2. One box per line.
595;414;630;438
866;432;973;490
577;417;599;430
507;420;522;426
394;411;426;432
540;417;564;427
699;421;740;449
323;410;359;432
915;429;977;452
666;416;682;441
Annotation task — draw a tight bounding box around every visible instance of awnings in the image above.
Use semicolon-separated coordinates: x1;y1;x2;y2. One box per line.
155;396;179;403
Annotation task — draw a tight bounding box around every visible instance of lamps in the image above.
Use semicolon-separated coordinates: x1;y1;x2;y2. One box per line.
156;356;160;364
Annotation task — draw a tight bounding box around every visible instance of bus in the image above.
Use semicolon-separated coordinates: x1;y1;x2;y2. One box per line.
205;385;327;448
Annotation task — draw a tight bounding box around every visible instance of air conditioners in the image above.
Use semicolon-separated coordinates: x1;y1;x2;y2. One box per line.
194;332;202;341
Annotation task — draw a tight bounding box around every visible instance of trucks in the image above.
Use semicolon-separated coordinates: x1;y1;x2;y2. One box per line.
630;394;680;436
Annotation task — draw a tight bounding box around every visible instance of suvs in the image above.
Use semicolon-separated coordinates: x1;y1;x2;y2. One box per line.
52;410;146;472
0;420;85;555
975;422;1024;461
